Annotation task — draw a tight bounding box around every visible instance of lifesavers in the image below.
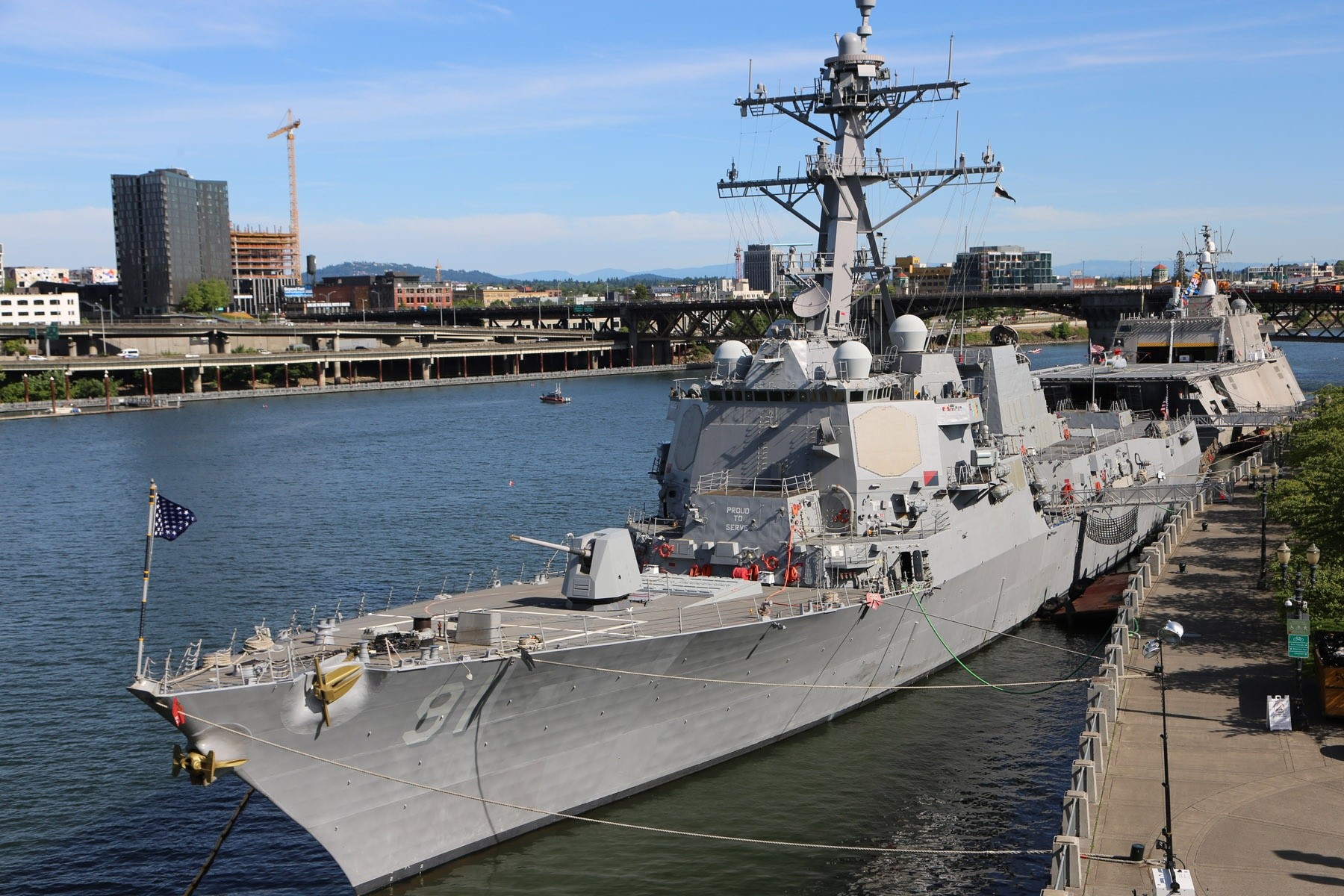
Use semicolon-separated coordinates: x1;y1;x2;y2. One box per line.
1064;429;1068;439
1064;483;1073;498
766;556;778;570
1097;482;1101;489
838;508;850;523
1021;445;1025;455
658;544;671;558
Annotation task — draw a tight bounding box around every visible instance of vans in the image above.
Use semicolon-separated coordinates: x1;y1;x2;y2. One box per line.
116;349;140;359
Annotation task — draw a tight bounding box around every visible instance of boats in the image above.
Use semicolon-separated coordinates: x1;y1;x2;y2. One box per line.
1031;223;1307;451
539;383;571;404
123;3;1211;896
1027;348;1042;354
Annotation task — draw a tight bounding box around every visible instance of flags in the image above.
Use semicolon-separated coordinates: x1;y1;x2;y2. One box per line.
1181;268;1203;307
1092;344;1105;353
154;493;197;541
1160;395;1169;419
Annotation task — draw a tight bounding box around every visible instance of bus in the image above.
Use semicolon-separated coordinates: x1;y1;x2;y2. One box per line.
288;344;311;353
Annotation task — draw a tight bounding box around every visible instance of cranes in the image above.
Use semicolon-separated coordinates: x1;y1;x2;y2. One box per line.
266;107;302;288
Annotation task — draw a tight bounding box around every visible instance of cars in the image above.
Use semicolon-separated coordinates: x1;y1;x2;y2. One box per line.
185;353;200;358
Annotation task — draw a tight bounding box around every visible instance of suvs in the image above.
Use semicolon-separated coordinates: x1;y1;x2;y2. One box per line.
355;346;369;351
319;346;333;352
24;354;48;362
259;350;273;355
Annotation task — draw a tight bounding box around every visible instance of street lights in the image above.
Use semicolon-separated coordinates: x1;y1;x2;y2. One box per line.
1276;539;1322;728
317;290;380;324
1249;460;1280;589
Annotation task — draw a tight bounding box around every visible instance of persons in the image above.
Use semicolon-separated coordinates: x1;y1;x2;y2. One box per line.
1255;401;1261;413
